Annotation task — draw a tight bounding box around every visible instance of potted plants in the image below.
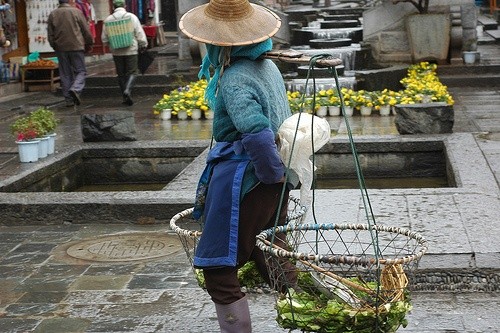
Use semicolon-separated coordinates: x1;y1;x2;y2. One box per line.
462;38;479;63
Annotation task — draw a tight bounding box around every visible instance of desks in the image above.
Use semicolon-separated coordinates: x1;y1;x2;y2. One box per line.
21;65;60;92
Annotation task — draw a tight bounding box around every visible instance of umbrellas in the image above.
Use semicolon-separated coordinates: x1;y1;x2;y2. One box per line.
138;46;155;77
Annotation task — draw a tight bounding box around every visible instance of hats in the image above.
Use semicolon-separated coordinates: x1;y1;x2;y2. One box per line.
179;0;282;46
113;0;125;7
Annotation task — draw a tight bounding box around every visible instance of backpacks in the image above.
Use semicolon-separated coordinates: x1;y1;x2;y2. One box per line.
105;13;133;49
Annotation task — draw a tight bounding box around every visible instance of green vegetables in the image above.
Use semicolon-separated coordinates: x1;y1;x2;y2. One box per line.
195;261;265;289
275;271;412;333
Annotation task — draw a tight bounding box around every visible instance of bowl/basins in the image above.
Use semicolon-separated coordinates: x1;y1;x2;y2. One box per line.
142;26;158;37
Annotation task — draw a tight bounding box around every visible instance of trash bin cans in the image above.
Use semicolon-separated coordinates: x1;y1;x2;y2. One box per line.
144;25;158;48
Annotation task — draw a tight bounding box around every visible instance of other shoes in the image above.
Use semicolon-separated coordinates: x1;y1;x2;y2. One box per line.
68;89;80;105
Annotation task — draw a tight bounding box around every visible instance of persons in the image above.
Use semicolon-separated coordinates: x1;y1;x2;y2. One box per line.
0;14;11;47
178;0;300;333
101;0;149;106
48;0;93;109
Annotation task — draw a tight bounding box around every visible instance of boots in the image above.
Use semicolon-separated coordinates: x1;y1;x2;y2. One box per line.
215;292;251;333
124;74;137;106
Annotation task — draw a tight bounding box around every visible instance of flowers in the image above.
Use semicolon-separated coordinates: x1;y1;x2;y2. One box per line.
153;77;212;116
288;62;454;113
11;107;61;141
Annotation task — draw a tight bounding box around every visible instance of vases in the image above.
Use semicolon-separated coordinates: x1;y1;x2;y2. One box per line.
191;109;201;120
421;95;432;103
15;132;57;163
360;106;372;116
316;106;327;117
328;105;340;116
379;104;390;116
178;109;188;119
160;109;172;120
342;106;354;116
205;109;214;119
